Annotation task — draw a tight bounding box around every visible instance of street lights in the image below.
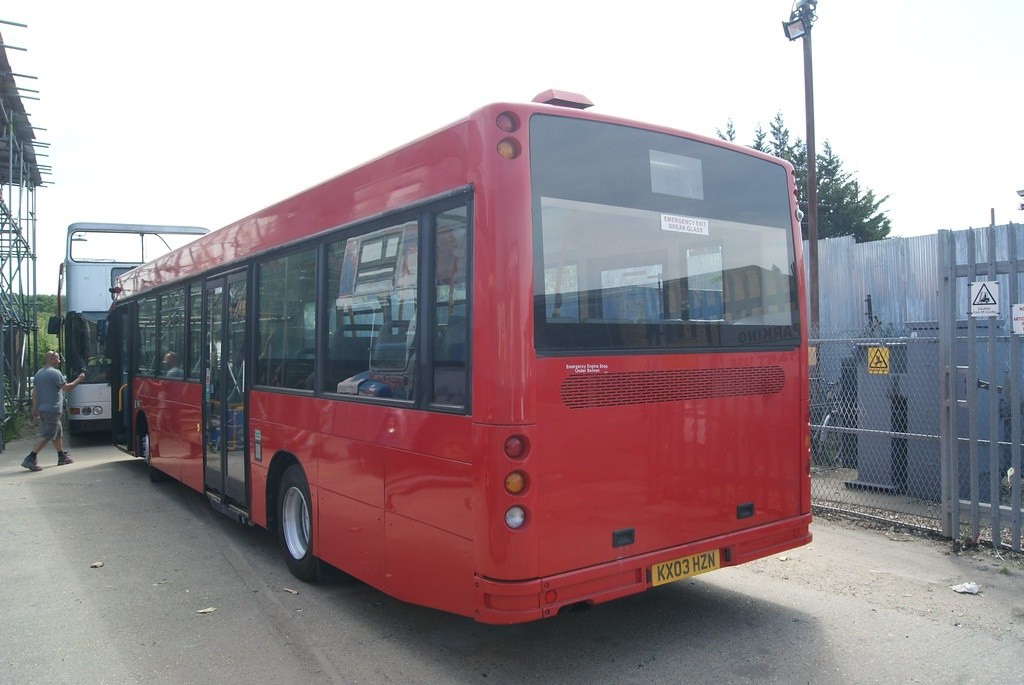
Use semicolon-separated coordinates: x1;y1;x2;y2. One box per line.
781;0;824;422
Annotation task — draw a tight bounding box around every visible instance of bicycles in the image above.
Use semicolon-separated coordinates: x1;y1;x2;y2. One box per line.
811;377;845;463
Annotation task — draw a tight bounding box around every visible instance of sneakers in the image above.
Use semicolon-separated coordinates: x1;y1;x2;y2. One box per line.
21;455;42;470
58;451;73;465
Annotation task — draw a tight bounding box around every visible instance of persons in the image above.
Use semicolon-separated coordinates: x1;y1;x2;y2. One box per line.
163;352;183;377
144;377;175;439
21;350;85;471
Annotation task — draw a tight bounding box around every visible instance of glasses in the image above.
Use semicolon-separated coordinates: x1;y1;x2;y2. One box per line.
50;350;54;359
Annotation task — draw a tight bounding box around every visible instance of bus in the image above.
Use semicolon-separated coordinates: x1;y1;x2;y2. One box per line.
96;91;813;623
48;222;211;436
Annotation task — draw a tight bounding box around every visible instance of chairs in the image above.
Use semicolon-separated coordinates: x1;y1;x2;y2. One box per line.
291;321;446;382
